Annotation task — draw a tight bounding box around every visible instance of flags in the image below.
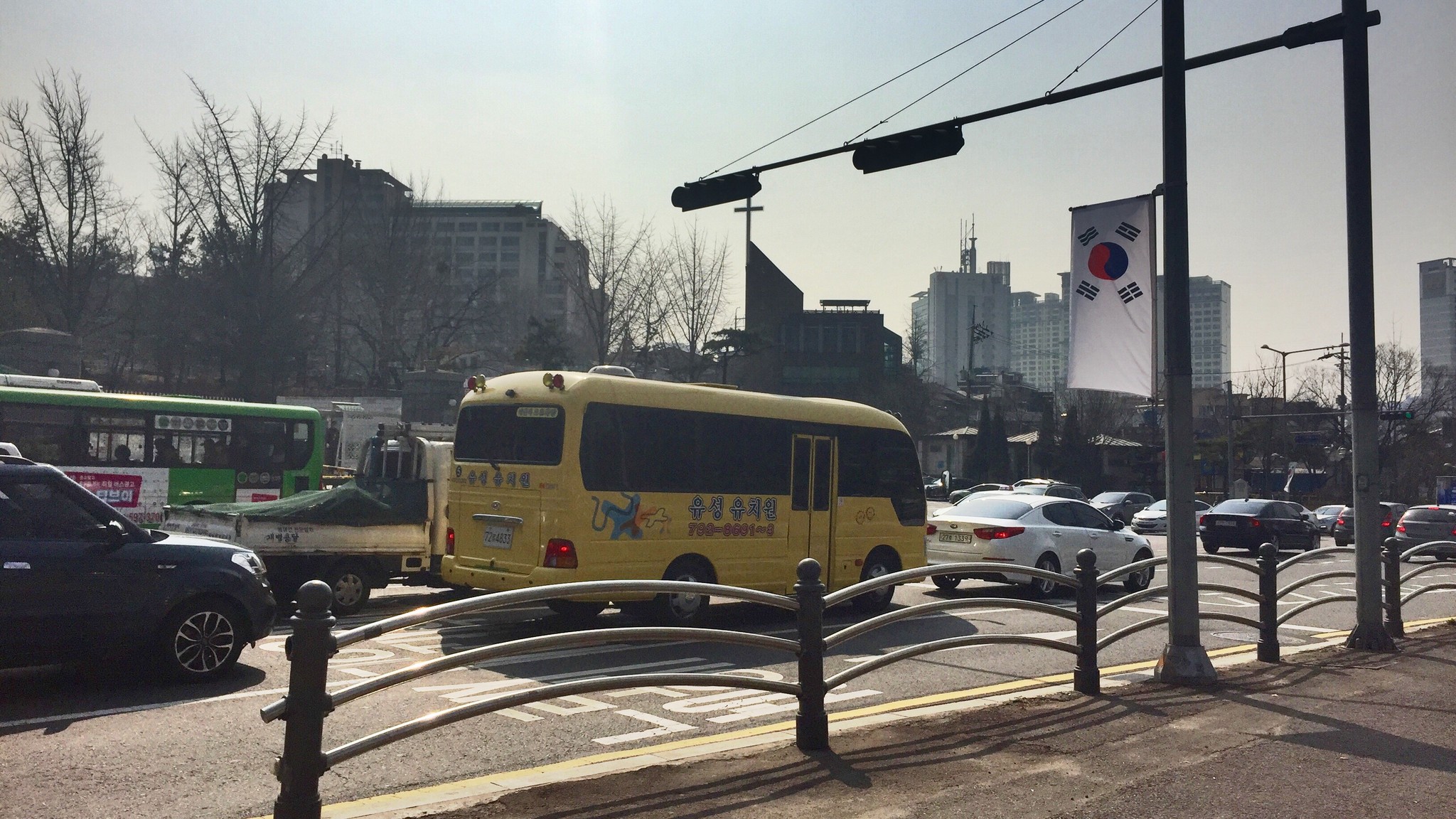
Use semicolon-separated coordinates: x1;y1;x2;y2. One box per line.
1068;195;1155;398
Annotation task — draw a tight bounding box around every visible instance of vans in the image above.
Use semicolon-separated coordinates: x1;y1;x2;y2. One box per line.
0;442;22;501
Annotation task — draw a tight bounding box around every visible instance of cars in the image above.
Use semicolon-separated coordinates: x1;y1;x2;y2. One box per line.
1282;501;1321;529
1395;504;1456;562
919;471;1090;519
1087;491;1156;526
924;494;1155;600
1130;499;1214;535
1312;505;1346;537
1199;498;1321;557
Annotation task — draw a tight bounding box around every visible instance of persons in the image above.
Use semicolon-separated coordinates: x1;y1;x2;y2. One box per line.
113;443;134;467
202;439;229;468
153;437;183;468
324;421;340;474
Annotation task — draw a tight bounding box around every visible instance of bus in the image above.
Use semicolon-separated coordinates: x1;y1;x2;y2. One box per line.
442;365;928;628
0;373;325;537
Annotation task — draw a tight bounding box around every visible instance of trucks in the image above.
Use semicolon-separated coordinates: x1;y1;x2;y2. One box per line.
157;436;456;617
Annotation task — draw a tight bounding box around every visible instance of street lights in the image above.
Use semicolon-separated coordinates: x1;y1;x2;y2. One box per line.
1261;343;1350;432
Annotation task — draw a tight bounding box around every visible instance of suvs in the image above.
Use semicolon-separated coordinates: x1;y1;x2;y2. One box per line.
0;455;279;681
1333;502;1411;546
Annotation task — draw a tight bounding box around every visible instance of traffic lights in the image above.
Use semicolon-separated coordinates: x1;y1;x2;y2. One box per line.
852;126;965;175
1379;410;1415;419
671;173;762;212
